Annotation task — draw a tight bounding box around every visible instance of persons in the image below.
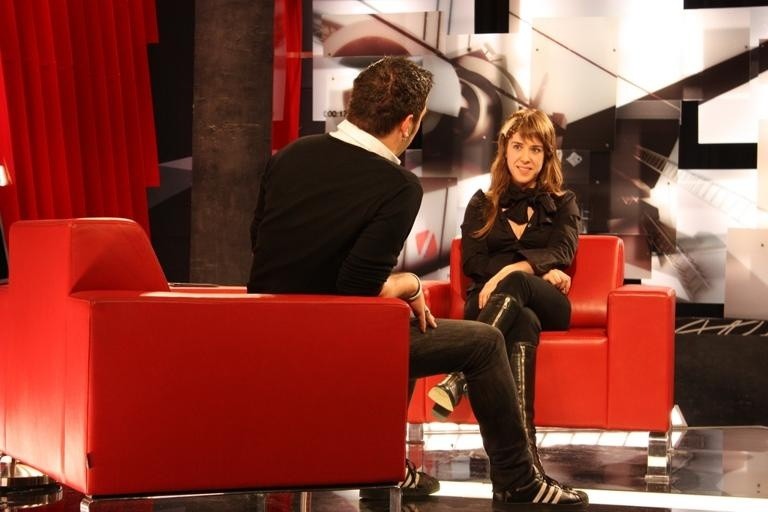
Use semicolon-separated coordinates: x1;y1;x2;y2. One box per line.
428;107;579;494
246;56;590;510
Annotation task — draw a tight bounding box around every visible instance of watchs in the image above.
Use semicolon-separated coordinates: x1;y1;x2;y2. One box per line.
408;273;421;301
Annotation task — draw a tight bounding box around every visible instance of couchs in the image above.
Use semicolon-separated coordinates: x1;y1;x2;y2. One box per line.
406;236;675;486
1;220;412;512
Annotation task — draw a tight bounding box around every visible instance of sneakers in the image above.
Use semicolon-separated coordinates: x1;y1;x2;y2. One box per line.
493;467;590;511
361;460;440;498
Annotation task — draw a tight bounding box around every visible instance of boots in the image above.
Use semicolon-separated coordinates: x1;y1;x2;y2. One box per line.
428;295;520;417
506;345;555;483
